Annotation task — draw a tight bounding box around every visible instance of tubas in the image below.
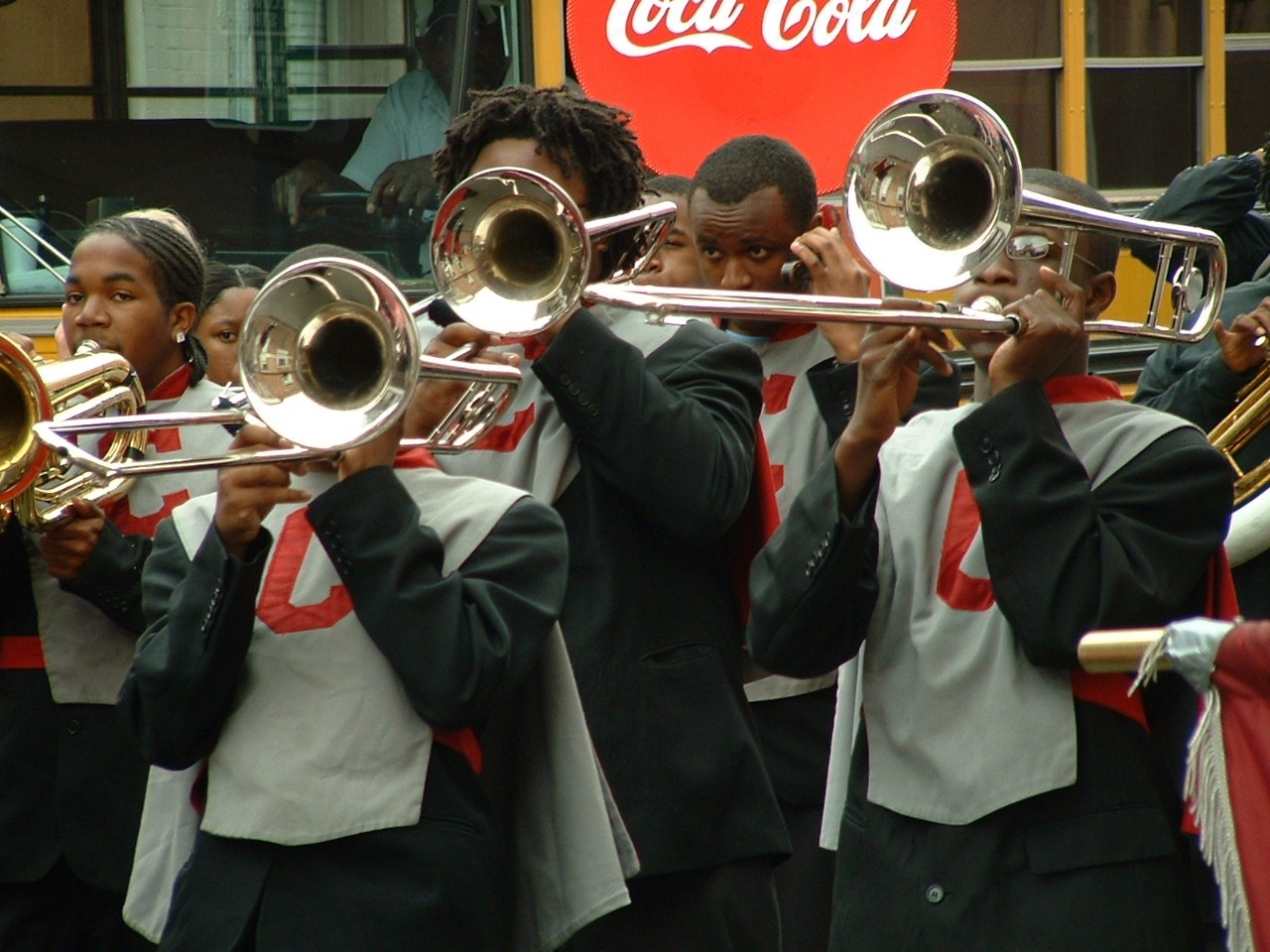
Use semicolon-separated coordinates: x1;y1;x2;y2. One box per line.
1206;335;1270;507
0;334;151;530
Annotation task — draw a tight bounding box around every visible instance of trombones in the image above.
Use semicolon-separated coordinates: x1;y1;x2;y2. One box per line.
581;89;1228;345
31;256;523;480
209;165;676;437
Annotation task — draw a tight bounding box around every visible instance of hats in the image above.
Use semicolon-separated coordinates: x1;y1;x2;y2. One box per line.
429;6;458;32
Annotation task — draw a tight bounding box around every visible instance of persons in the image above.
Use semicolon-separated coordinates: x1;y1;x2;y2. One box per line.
119;244;569;950
0;212;250;952
379;84;790;952
872;158;897;182
54;208;205;363
1133;269;1270;622
269;0;582;277
1121;130;1270;284
444;207;464;269
627;173;706;290
188;263;272;391
741;172;1233;951
674;135;961;951
259;322;278;346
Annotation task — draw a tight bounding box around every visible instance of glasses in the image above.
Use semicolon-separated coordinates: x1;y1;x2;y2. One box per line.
1005;234;1103;273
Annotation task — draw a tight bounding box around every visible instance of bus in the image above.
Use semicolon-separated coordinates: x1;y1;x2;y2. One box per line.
0;0;1270;410
